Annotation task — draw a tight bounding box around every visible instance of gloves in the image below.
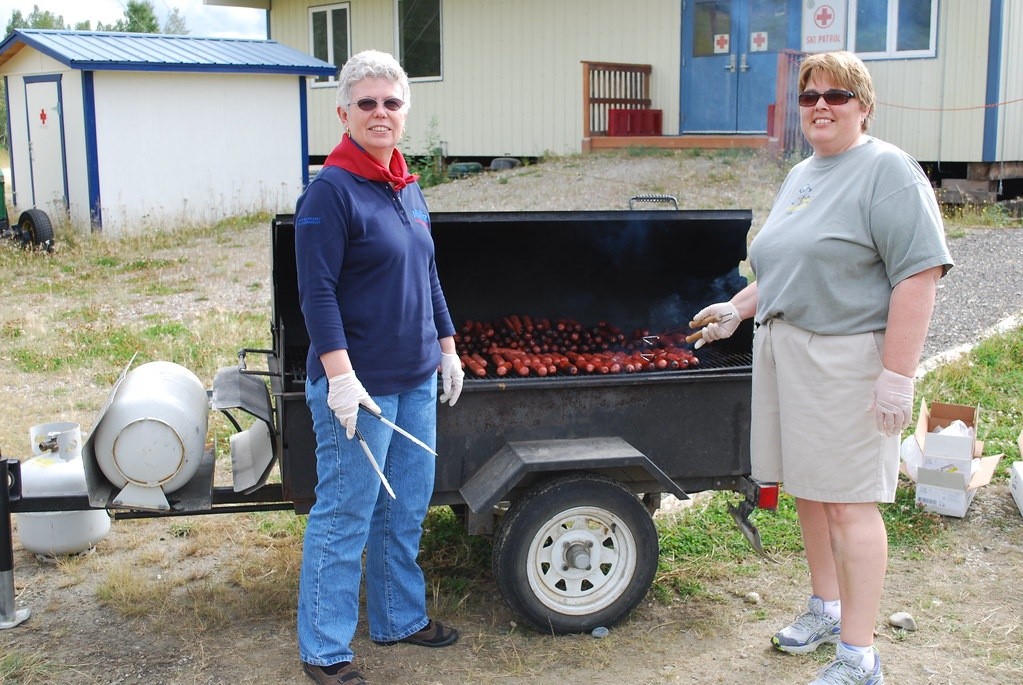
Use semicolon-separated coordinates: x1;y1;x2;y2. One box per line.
693;301;742;350
327;371;381;440
439;352;465;406
865;368;914;437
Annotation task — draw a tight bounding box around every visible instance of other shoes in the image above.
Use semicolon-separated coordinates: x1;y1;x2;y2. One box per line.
375;619;459;647
304;660;369;685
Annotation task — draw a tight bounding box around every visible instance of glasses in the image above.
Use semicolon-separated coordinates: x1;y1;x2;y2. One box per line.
348;98;406;112
799;88;856;107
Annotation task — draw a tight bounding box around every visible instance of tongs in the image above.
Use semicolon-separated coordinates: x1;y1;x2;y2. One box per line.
640;312;735;362
354;404;438;498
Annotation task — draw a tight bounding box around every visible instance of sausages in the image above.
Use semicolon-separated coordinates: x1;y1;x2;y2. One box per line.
437;312;698;377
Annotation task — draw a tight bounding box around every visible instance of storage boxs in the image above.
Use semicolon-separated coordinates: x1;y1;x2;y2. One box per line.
1010;460;1023;518
900;396;1005;517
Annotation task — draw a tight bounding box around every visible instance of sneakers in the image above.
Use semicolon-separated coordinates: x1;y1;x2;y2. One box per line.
771;596;841;655
808;641;884;685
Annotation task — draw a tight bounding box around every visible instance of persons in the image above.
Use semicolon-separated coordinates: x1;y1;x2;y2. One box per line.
293;47;464;684
688;51;955;685
